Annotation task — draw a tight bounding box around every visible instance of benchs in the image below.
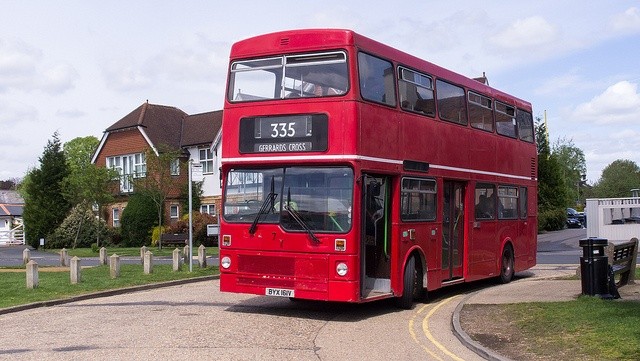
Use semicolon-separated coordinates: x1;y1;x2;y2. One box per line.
603;237;638;290
158;233;186;248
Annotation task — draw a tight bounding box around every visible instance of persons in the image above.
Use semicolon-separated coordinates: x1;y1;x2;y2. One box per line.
475;193;504;219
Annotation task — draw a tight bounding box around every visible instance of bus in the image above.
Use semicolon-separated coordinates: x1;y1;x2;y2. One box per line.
220;30;538;310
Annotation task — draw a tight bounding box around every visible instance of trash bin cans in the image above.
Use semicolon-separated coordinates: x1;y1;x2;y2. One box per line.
580;236;609;297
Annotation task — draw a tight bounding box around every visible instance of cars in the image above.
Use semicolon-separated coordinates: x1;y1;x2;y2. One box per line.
567;207;584;222
566;216;581;228
